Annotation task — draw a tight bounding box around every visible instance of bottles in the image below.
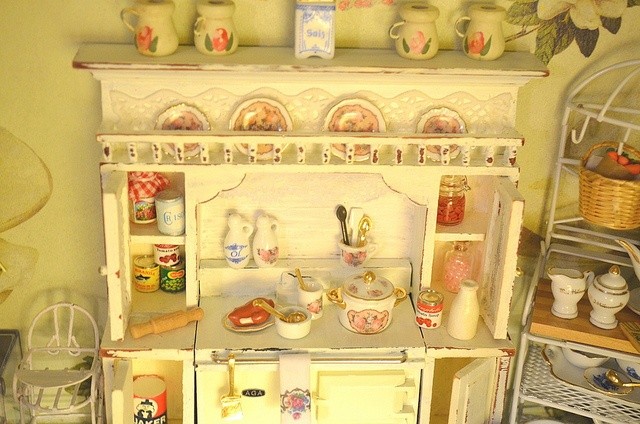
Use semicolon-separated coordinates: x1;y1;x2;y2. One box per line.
447;280;480;340
442;241;474;293
224;214;252;269
252;216;280;268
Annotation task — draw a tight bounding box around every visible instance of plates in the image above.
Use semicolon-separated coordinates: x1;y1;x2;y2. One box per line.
584;367;633;396
414;105;469;162
542;344;640;404
228;98;294;160
154;101;210;160
223;303;281;333
322;98;386;162
524;417;563;424
627;287;640;315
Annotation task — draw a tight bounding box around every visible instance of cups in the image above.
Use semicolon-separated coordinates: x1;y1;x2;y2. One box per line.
297;283;324;320
547;266;595;319
120;0;179;57
388;3;440;60
192;0;239;56
588;265;629;330
338;237;376;269
455;3;506;61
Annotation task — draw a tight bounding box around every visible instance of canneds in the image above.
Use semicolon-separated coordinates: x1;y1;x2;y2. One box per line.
127;171;172;225
159;259;186;292
153;244;181;266
133;255;160;293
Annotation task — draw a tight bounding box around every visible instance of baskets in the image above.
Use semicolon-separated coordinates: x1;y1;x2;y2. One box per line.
578;141;640;231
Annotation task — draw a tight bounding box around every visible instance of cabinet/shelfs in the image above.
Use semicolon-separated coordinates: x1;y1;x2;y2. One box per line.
101;353;511;424
510;61;639;423
73;44;551;352
13;300;100;424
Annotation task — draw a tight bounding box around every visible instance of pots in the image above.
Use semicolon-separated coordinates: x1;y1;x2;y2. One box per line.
325;270;407;335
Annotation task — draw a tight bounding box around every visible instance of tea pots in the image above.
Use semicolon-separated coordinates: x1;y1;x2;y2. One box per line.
615;238;640;281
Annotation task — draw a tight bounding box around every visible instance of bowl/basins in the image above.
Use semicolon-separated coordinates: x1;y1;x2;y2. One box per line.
562;347;611;369
275;305;312;339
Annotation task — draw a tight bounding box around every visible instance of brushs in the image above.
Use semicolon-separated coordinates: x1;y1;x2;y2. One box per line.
219;352;247;424
